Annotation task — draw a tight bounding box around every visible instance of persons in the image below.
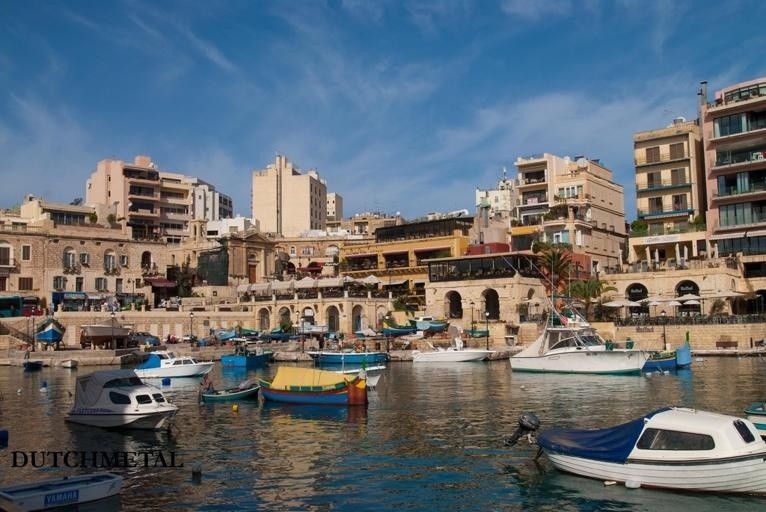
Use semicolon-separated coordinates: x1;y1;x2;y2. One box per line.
24;344;32;358
200;372;214;395
175;295;181;307
167;332;178;344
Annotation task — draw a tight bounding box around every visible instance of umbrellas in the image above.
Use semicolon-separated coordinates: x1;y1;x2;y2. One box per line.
523;287;747;315
300;274;382;284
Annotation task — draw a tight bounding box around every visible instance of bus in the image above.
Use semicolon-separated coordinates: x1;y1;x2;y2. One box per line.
0;296;22;318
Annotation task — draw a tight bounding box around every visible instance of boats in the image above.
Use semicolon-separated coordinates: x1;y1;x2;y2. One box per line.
0;473;122;511
744;402;766;438
501;408;766;500
